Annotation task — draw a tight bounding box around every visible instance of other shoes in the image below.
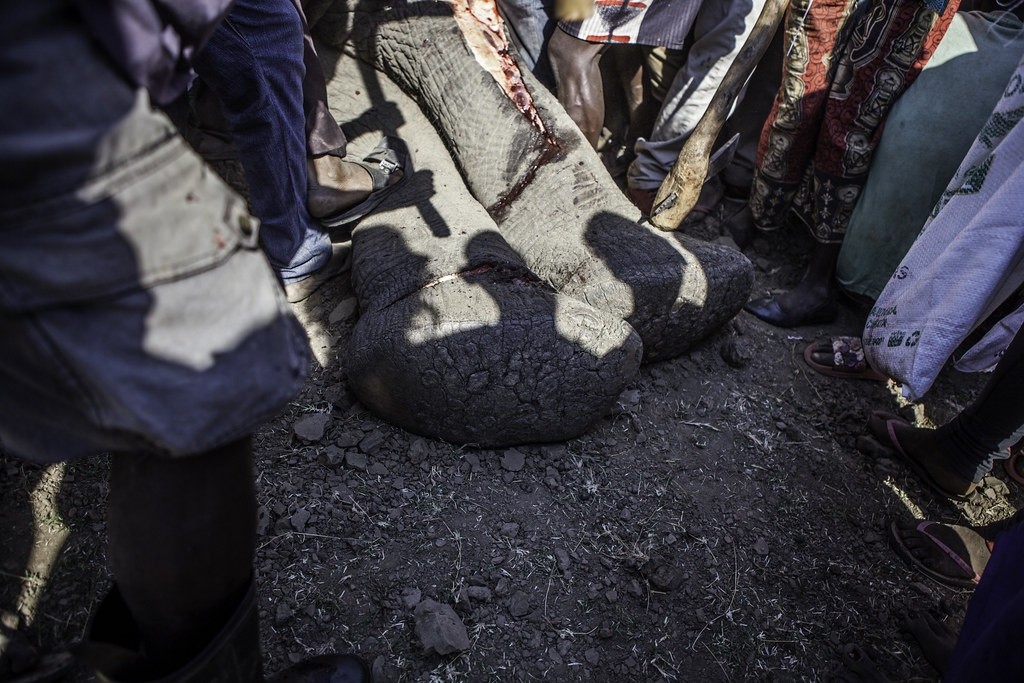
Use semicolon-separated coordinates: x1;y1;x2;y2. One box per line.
615;130;810;257
271;240;360;304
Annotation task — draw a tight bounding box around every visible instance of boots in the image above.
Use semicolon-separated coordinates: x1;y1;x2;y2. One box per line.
87;567;371;683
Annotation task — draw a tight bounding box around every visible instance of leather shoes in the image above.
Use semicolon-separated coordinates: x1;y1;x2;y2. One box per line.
744;293;835;328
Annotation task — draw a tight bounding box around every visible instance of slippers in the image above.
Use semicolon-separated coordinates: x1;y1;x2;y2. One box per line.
888;517;982;588
864;407;977;502
317;135;414;229
804;336;891;382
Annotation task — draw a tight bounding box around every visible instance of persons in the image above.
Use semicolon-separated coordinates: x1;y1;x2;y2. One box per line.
190;0;412;228
746;0;1024;683
547;0;654;175
637;0;703;110
191;0;354;303
0;0;372;683
626;0;767;223
